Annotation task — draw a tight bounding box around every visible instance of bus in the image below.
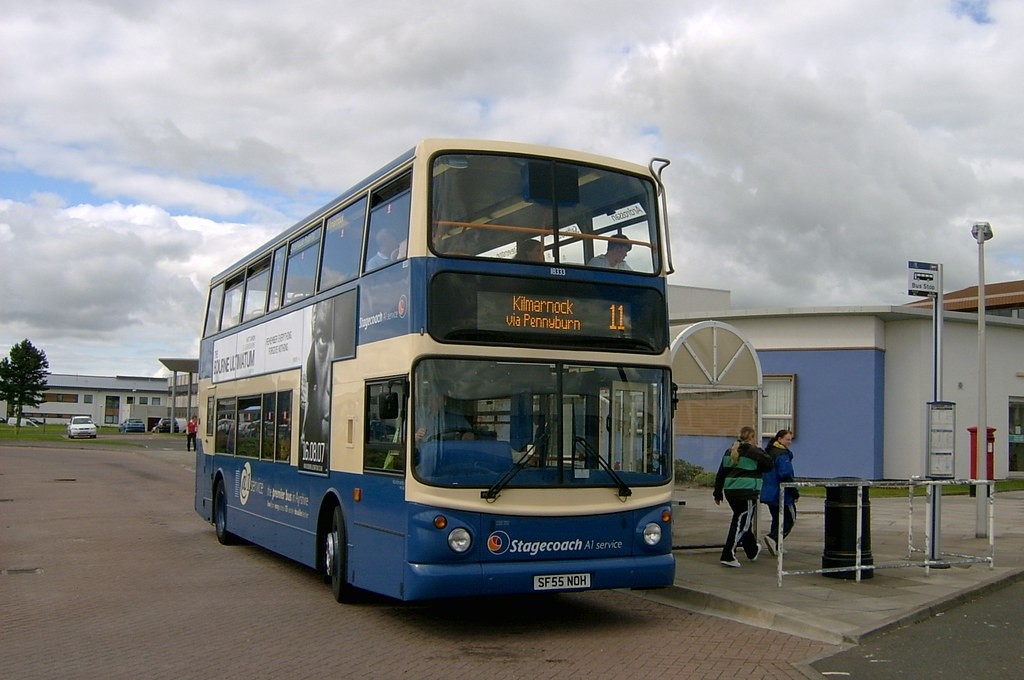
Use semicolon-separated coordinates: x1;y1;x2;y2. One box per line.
191;135;685;607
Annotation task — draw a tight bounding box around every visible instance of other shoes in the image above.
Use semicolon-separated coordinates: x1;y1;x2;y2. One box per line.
751;543;761;561
720;559;740;568
764;536;776;557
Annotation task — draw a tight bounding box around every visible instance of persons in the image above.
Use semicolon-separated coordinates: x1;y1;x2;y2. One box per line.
513;239;540;261
585;234;633;269
186;415;197;452
415;391;475;441
304;291;355;471
760;430;800;556
367;228;400;272
712;426;774;568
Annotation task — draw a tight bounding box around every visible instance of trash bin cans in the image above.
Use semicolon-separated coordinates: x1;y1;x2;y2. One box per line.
820;475;877;578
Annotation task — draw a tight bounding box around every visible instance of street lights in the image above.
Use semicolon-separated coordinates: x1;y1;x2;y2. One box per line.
972;220;995;540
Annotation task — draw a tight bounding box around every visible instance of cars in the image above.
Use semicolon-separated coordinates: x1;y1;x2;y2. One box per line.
151;417;180;433
30;418;44;425
0;416;6;423
119;418;145;434
64;415;98;439
7;416;39;428
